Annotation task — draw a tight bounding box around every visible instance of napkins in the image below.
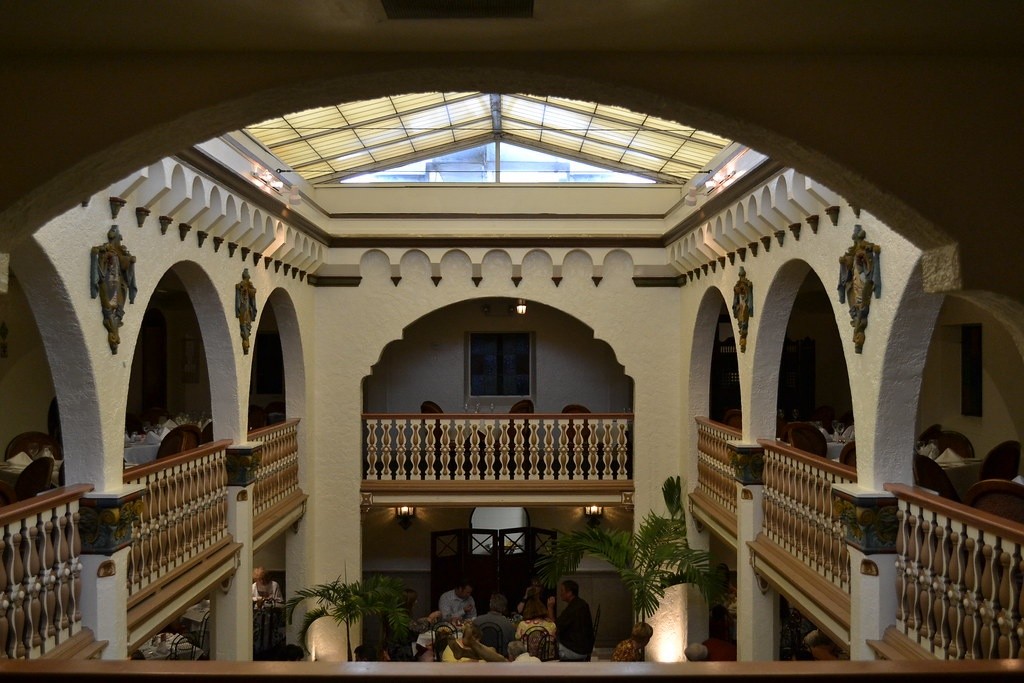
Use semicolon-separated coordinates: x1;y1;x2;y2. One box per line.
6;448;55;468
818;427;832;442
933;447;964;465
143;419;177;444
156;640;167;654
842;425;854;441
1011;475;1024;487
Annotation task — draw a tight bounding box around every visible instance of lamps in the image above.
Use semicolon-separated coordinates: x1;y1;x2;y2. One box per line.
515;299;527;314
283;185;301;204
685;185;705;206
584;506;603;529
396;507;416;530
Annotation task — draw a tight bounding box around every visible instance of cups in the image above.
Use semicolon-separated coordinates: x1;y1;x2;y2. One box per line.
778;408;784;419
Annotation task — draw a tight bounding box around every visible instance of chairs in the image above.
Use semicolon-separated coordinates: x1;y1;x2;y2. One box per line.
479;622;503;656
723;408;742;430
381;612;390;643
913;430;1024;526
560;405;615;479
248;401;285;431
0;457;65;510
166;599;282;660
156;420;213;460
431;621;457;662
780;608;818;661
522;627;559;661
420;400;464;465
5;431;54;461
563;604;602;662
775;404;856;467
489;399;534;478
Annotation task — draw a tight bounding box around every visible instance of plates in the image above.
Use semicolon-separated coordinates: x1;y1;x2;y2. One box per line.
964;457;982;463
179;642;193;650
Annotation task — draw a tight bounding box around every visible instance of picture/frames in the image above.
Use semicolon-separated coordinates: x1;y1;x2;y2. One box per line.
465;329;536;402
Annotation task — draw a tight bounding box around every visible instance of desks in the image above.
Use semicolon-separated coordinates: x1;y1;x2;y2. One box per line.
416;624;463;649
827;442;847;458
124;444;161;466
134;631;205;659
0;458;64;485
942;458;983;500
182;601;286;655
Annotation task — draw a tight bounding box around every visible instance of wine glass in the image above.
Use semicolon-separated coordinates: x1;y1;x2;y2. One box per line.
792;408;799;421
132;431;137;443
837;423;844;438
144;424;164;436
465;402;495;414
29;442;42;461
832;420;839;442
915;439;938;460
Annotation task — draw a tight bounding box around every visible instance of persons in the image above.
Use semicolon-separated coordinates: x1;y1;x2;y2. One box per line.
709;605;734;641
285;644;304;661
439;578;477;621
508;641;542;663
547;580;593;660
443;624;511;662
514;597;557;658
716;563;736;594
517;575;553;616
803;628;828;649
385;591;442;661
473;594;516;658
354;645;378;662
253;568;283;603
611;622;653;662
